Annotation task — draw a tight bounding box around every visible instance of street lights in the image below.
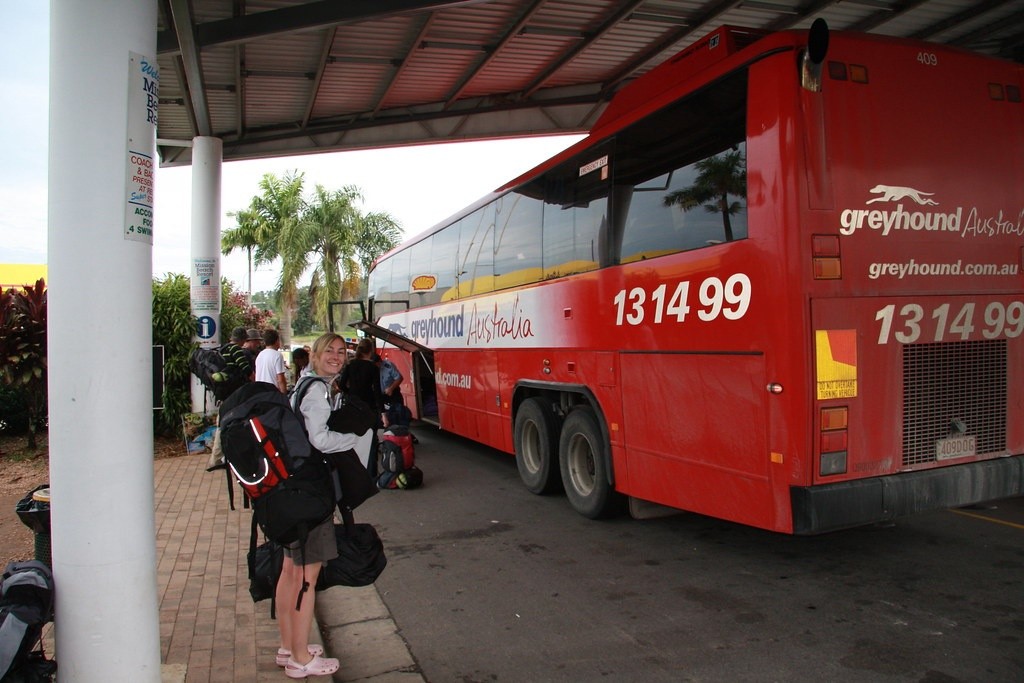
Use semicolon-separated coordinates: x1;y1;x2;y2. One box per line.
227;211;252;304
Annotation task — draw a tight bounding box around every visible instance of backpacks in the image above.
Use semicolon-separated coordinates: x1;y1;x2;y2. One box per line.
378;423;423;490
189;343;245;400
0;558;55;683
221;376;336;547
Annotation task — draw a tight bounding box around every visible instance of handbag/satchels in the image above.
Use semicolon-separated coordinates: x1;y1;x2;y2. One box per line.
247;498;386;603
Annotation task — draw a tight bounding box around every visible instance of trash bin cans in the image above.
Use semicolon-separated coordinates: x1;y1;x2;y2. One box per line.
32;487;52;573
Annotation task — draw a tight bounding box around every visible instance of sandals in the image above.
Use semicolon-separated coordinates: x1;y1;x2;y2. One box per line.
285;653;340;678
276;644;323;665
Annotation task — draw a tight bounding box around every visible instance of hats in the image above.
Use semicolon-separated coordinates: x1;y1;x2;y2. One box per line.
371;354;380;362
230;327;249;341
247;329;265;341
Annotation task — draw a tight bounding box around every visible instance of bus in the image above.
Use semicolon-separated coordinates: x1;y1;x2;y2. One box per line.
329;18;1024;534
